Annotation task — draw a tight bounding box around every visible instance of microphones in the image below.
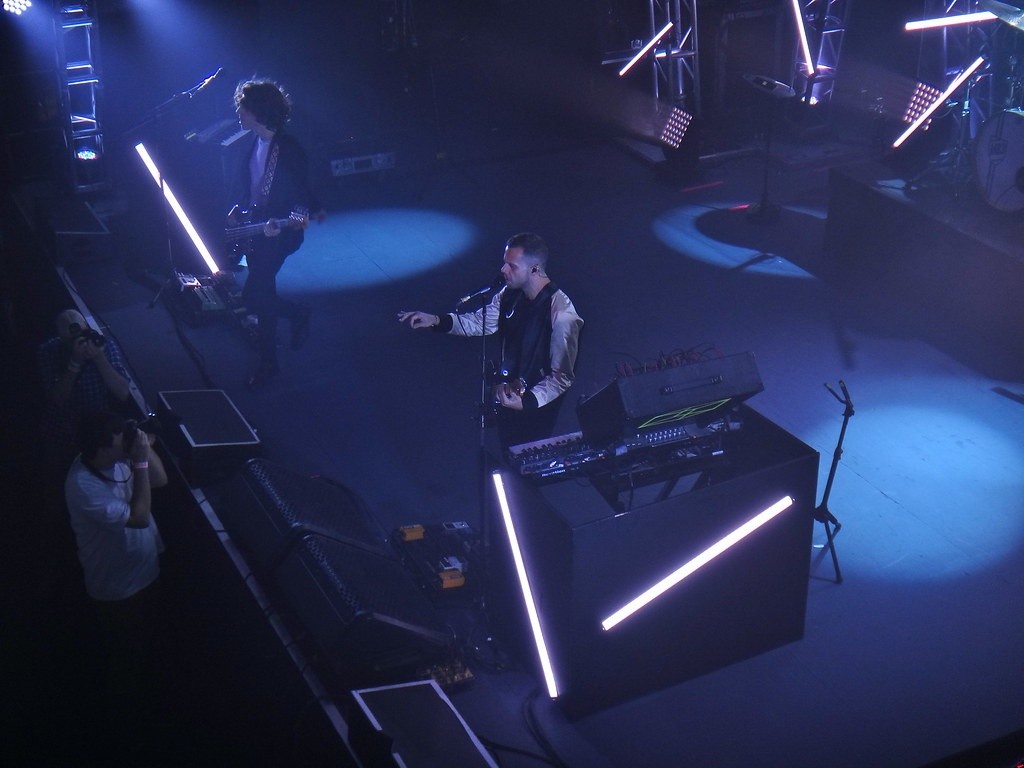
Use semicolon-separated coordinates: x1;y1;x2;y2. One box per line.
460;277;505;304
192;66;224;96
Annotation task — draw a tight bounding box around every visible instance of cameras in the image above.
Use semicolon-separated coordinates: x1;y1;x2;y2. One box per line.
124;410;163;450
76;329;105;348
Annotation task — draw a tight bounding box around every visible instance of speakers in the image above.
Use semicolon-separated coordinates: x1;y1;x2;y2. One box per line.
220;458;456;682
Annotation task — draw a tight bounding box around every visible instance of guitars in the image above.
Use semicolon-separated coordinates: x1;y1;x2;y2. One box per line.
224;204;310;266
470;359;528;424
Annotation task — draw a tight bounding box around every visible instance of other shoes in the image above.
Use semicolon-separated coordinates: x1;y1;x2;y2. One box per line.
289;302;312;350
242;358;273;391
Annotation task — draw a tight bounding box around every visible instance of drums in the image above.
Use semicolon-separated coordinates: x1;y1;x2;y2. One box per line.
971;107;1024;212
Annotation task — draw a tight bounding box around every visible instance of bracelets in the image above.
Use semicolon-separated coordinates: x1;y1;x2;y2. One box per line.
132;460;148;469
68;361;80;371
431;315;438;326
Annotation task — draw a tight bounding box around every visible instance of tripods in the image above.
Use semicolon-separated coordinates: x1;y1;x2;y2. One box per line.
813;379;852;583
907;77;975;204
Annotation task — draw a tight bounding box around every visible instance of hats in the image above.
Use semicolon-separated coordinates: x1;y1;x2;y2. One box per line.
56;309;89;338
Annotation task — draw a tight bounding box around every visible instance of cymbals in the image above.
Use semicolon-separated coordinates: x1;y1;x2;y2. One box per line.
979;0;1024;33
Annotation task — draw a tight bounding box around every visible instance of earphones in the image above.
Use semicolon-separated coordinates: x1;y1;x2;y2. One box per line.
532;268;536;273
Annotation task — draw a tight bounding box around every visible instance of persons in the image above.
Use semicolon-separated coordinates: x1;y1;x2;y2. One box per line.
395;229;582;464
35;309;169;758
180;82;316;388
